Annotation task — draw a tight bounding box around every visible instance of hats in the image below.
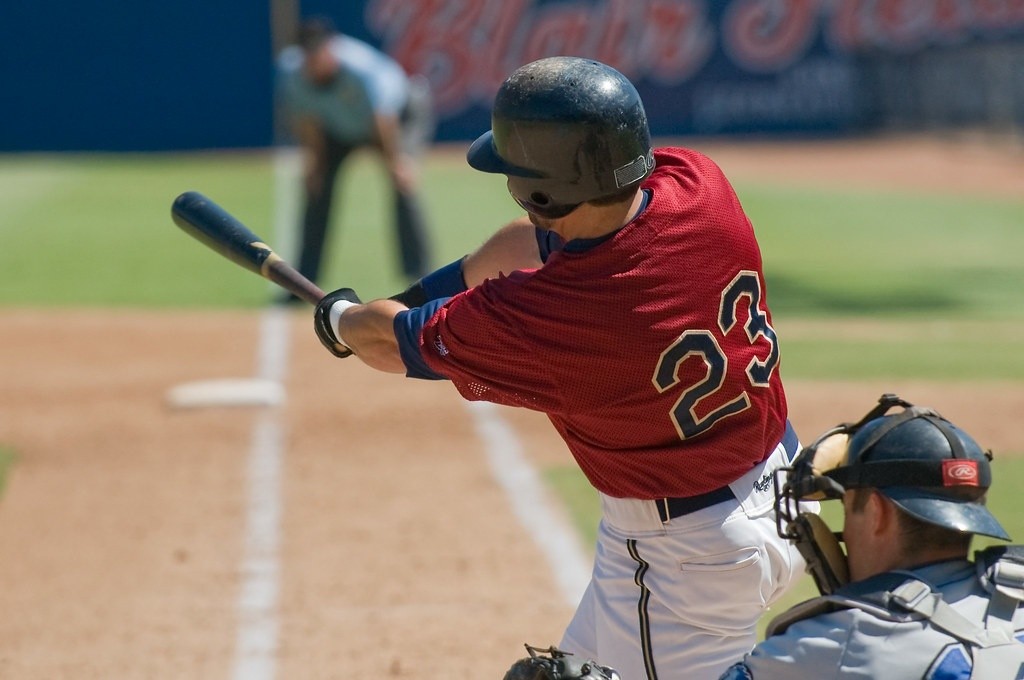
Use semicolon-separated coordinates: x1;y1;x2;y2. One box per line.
838;411;1012;542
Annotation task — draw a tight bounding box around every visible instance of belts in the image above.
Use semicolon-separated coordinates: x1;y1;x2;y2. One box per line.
655;421;798;524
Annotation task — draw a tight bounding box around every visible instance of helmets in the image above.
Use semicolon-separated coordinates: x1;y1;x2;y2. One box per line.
467;57;656;219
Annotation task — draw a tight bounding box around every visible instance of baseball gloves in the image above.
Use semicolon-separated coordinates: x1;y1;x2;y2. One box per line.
499;653;622;680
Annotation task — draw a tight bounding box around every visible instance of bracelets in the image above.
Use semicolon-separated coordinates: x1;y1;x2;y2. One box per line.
329;300;360;350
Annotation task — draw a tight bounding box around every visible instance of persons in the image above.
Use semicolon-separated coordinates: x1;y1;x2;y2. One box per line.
504;411;1023;679
313;56;822;679
275;23;431;302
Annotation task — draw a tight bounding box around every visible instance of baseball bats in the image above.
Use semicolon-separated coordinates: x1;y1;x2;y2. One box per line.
169;190;327;308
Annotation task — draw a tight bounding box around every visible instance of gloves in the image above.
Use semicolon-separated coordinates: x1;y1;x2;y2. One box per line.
314;288;363;358
